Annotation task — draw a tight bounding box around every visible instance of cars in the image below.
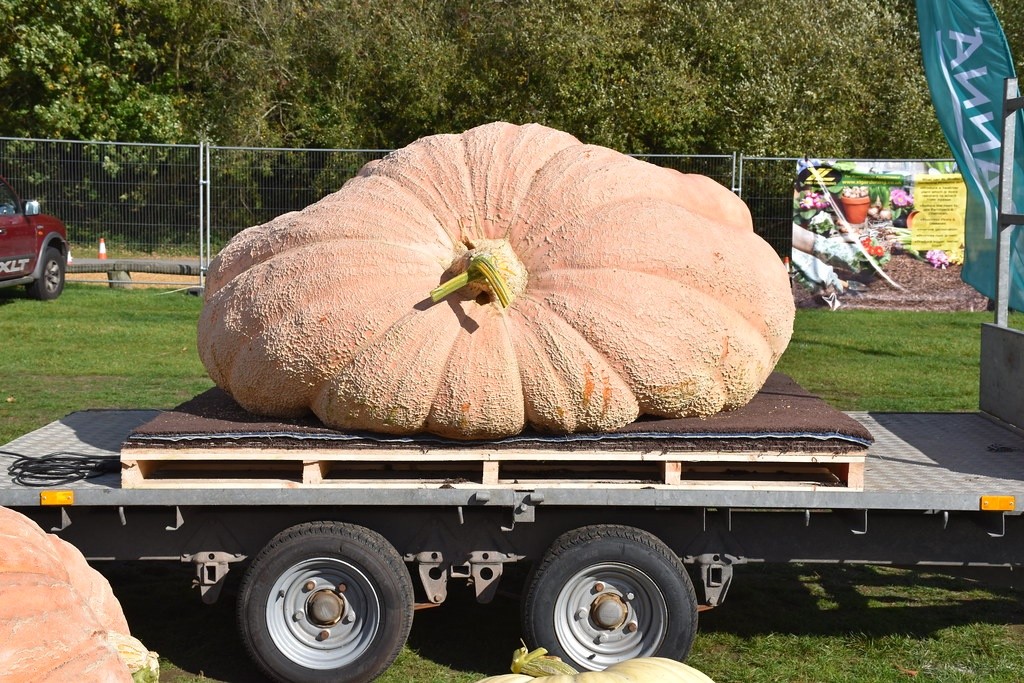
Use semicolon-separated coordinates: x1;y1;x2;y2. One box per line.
0;175;68;300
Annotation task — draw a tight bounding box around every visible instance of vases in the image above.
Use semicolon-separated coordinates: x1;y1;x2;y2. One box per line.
800;216;810;229
812;225;831;238
907;211;920;229
893;212;907;227
840;193;870;223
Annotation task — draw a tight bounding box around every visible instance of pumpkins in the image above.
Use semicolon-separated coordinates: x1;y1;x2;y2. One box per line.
472;657;714;683
199;123;795;441
0;506;152;683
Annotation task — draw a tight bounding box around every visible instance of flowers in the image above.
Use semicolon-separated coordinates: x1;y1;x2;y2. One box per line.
842;186;868;198
810;211;835;234
889;189;914;220
793;190;830;220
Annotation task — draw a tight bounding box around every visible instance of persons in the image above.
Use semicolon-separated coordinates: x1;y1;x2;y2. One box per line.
792;219;891;308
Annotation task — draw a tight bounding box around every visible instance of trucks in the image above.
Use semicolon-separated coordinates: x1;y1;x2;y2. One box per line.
0;78;1023;683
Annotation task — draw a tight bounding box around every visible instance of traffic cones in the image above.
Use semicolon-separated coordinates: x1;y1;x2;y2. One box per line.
98;238;107;261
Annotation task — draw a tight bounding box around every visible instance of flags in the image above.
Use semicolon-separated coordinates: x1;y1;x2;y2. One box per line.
917;0;1024;314
